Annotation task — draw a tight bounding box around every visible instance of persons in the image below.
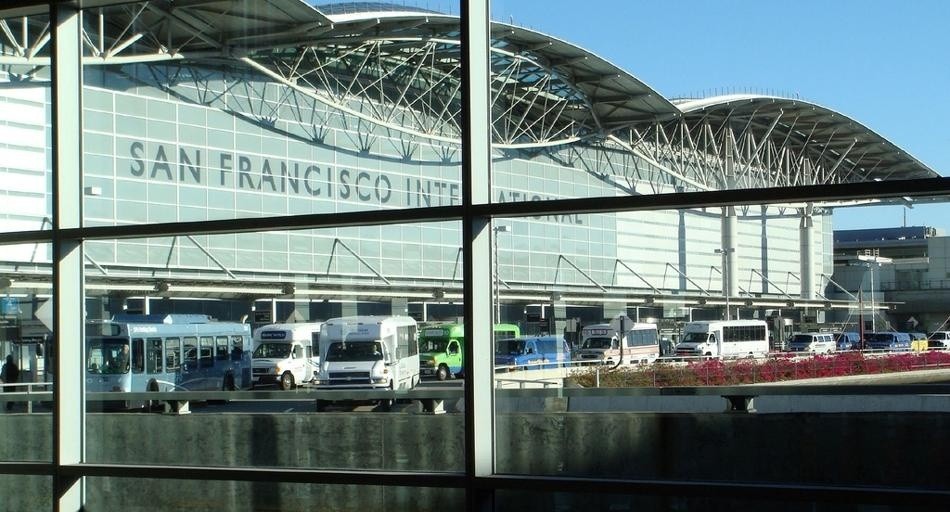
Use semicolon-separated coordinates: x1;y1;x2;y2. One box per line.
370;344;380;357
1;355;19;412
112;344;129;372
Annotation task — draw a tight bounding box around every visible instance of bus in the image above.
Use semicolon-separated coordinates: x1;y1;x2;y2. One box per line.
37;312;253;412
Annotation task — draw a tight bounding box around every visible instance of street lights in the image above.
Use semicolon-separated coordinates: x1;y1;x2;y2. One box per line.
857;253;894;331
715;248;735;320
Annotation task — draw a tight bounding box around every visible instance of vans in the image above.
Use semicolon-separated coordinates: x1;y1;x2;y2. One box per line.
497;335;571;373
783;330;950;352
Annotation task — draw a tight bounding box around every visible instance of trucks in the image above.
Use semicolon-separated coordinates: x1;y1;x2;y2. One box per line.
671;319;770;362
579;322;661;370
313;315;421;413
417;323;519;381
251;322;329;389
